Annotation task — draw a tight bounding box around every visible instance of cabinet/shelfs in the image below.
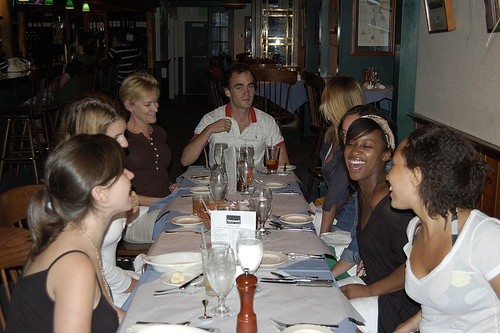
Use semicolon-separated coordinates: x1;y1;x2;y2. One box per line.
16;0;160;71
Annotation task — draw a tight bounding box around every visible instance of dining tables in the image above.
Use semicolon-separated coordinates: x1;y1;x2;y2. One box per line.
117;166;357;333
254;75;308;135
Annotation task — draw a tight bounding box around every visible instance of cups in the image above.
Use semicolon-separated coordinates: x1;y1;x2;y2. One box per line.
201;242;231;297
192;195;210;214
265;146;280;172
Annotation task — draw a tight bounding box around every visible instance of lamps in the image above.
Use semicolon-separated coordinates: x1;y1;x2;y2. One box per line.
82;0;90;12
65;0;74;9
44;0;54;5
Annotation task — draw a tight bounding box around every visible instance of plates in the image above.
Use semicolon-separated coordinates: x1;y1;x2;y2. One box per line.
141;252;204;272
188;186;212;194
128;324;209;333
260;250;288;266
160;271;204;286
281;324;336;333
279;214;313;224
278;165;297;171
264;181;288;188
195;176;210;182
171;215;203;226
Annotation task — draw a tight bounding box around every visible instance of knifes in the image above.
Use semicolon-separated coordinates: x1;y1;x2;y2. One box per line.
260;281;334;287
261;278;334;283
180;273;204;289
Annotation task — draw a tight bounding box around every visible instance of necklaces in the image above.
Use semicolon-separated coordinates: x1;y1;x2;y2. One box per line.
70;224;121;325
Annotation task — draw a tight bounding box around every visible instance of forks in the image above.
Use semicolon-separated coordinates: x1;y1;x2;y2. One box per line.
181;195;211;198
271;272;319;279
286;253;325;260
271;318;339;329
153;291;196;296
136;321;190;325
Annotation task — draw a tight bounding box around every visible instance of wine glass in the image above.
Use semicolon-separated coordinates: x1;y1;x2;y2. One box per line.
238;158;254;195
236;231;263;293
240;145;255;160
210;172;229;209
214;143;228;171
208;248;236;318
253;187;273;234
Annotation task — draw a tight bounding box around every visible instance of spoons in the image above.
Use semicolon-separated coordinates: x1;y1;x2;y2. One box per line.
165;228;210;234
155;287;177;292
199;300;212;320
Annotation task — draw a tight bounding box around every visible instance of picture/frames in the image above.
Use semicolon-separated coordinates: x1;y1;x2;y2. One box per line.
350;0;397;56
424;0;457;34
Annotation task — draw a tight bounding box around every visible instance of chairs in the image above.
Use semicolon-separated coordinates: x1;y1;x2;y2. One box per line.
208;56;299;122
0;52;112;333
301;70;332;160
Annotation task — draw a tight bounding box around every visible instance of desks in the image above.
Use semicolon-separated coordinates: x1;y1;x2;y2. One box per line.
361;83;393;109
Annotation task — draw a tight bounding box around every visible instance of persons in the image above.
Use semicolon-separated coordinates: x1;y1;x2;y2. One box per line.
180;62;290;169
5;133;134;333
24;31;98;144
329;108;422;333
56;97;143;313
98;30;144;97
118;72;183;244
385;124;500;333
310;75;365;260
320;104;393;277
